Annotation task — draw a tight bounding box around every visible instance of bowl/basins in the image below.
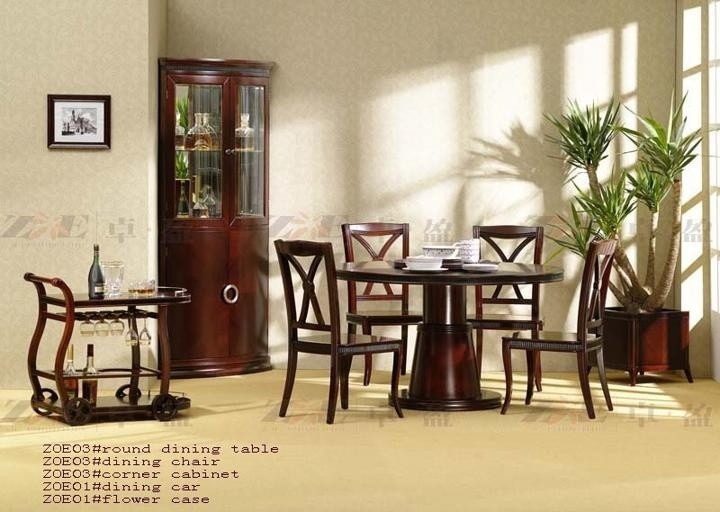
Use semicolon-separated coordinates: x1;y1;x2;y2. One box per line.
456;238;480;262
405;256;443;269
422;246;459;258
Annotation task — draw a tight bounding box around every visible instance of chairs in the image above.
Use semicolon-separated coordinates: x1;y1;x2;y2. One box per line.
465;224;543;392
274;239;404;424
341;222;423;385
501;236;618;419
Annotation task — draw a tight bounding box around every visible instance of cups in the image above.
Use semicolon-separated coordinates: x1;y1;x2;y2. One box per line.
127;279;158;297
100;260;126;297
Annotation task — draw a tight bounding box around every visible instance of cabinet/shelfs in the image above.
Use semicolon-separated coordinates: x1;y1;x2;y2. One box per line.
160;226;274;382
157;57;276;226
24;272;193;426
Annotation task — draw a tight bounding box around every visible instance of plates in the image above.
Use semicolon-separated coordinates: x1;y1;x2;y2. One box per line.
402;268;450;274
462;264;499;273
442;263;463;272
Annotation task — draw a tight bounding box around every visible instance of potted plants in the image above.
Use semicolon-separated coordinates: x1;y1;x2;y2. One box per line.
540;86;704;385
175;152;191;215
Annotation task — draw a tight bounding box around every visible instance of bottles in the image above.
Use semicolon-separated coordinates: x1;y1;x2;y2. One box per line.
81;342;98;407
192;179;217;219
177;179;189;214
235;112;256;150
174;111;185;152
184;112;219;150
88;242;105;301
62;343;79;403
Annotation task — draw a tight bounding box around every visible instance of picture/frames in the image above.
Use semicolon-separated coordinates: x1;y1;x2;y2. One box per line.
46;93;111;151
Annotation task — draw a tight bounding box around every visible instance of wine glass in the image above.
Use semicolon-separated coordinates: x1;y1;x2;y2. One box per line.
95;311;109;337
79;312;95;339
138;310;153;346
109;311;124;336
124;311;140;348
